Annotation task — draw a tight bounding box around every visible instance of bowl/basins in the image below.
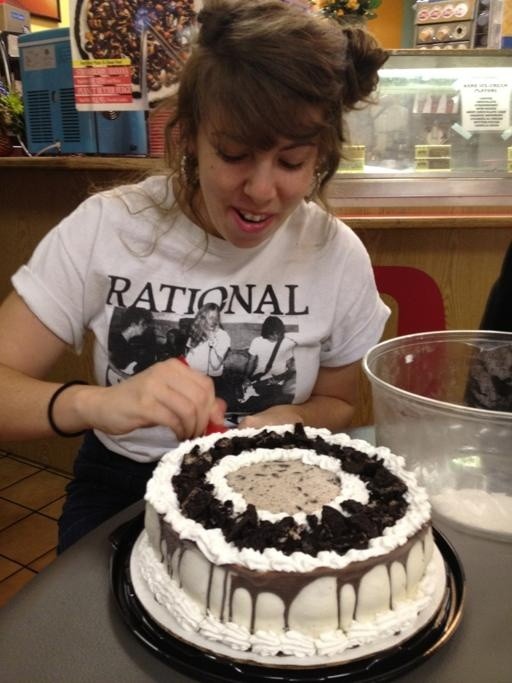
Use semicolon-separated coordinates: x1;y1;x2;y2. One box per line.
359;328;511;541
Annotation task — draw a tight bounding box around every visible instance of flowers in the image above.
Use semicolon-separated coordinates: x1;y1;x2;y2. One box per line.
305;0;384;26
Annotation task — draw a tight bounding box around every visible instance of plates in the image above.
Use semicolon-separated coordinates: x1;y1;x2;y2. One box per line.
106;503;467;683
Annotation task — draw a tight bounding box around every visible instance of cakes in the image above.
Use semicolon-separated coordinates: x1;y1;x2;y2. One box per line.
134;422;447;666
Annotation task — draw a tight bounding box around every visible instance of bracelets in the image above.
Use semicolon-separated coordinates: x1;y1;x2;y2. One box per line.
46;378;93;437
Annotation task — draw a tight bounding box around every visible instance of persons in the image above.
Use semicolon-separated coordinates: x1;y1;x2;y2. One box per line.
0;0;395;556
460;238;511;415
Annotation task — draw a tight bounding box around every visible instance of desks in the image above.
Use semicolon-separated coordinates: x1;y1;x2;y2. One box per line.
0;421;512;681
